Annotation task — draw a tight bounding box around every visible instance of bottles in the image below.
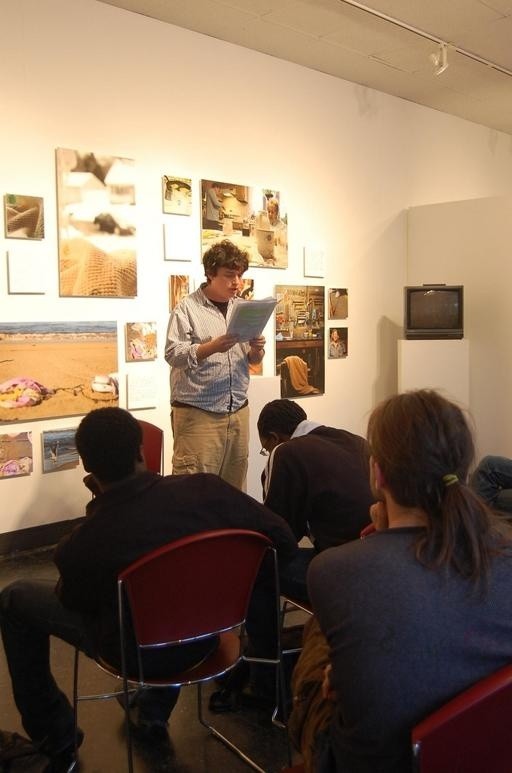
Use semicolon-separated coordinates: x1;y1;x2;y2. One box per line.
276;312;317;340
223;206;276;259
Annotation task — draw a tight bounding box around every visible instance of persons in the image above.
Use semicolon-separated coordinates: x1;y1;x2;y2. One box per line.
165;240;266;493
206;183;227;229
329;328;345;358
265;198;286;246
259;399;373;605
469;455;512;510
0;408;301;772
291;389;512;773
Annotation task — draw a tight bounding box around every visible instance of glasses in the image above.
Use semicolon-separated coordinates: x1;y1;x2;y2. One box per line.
258;435;274;457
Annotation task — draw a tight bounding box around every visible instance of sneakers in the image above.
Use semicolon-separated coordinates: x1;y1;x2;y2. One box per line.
114;680;142;727
208;673;277;714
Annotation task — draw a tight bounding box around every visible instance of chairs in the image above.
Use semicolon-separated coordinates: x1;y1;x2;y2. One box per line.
278;522;376;737
134;420;164;477
65;528;286;772
410;664;512;773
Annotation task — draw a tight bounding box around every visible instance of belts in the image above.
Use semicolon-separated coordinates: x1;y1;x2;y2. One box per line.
171;399;249;418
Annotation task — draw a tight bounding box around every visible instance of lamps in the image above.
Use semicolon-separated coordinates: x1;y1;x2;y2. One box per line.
428;42;450;79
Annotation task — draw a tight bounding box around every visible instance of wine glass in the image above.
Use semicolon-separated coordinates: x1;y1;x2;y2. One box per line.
143;327;156;359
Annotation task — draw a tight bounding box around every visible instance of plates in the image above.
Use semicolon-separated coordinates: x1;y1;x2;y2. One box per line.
203;230;223;253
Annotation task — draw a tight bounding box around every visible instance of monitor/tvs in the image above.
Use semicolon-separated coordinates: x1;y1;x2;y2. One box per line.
404;285;465;339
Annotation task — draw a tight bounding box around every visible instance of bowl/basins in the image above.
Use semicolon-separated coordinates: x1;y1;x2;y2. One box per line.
66;203;136;251
